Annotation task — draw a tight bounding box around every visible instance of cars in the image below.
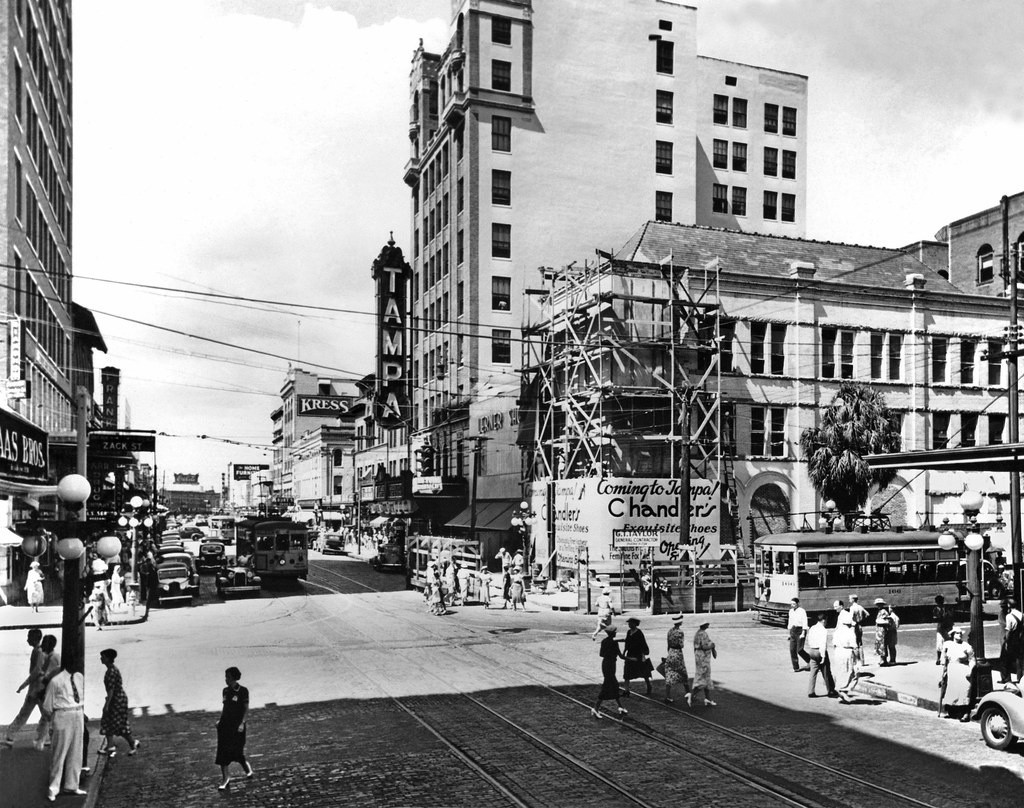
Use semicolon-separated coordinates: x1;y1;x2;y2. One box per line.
971;674;1024;750
954;558;1006;600
156;513;204;605
370;543;406;575
304;527;348;556
196;543;227;573
214;554;263;598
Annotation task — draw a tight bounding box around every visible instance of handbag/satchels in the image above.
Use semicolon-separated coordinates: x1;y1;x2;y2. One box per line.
657;657;667;678
832;627;859;649
636;655;654;677
711;642;717;659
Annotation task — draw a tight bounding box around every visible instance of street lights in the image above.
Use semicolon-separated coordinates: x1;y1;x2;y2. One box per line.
933;490;1007;669
16;473;132;751
818;499;840;534
509;502;536;592
110;491;169;588
294;505;348;527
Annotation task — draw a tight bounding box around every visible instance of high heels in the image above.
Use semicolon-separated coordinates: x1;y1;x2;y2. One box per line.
245;761;253;776
98;746;116;754
591;708;603;718
218;778;230;789
684;693;692;708
704;698;717;706
618;707;628;714
129;740;140;755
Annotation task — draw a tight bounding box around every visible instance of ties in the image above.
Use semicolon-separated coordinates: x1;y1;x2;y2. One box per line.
70;673;79;703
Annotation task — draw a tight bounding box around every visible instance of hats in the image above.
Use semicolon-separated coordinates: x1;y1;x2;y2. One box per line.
873;598;887;605
948;626;965;638
626;618;640;626
602;587;612;594
604;625;617;633
672;614;683;624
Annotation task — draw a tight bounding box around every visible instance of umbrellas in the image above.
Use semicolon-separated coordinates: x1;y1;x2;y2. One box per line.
985;545;1006;553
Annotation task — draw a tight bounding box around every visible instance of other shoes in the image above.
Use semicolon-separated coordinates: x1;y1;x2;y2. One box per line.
666;697;675;703
33;739;52;745
997;681;1008;683
81;766;91;772
828;692;838;697
0;739;14;746
809;693;819;698
791;669;799;672
63;788;87;795
48;795;56;801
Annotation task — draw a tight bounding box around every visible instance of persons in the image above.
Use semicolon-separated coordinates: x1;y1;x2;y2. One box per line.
621;617;654;698
886;604;901;631
185;496;576;617
832;617;863;704
785;597;813;673
805;612;838;699
588;623;629;720
998;598;1024;684
0;628;52;749
30;635;63;753
95;649;142;756
109;565;126;610
830;598;854;672
136;532;161;600
663;612;692;703
38;651;89;802
871;595;892;666
684;616;719;708
214;667;253;789
23;561;46;614
89;583;107;633
994;551;1006;570
936;625;978;722
847;594;870;666
931;595;957;665
591;586;618;640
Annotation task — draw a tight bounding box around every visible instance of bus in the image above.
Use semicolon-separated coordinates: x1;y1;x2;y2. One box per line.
749;525;985;627
236;518;309;585
208;517;235;545
